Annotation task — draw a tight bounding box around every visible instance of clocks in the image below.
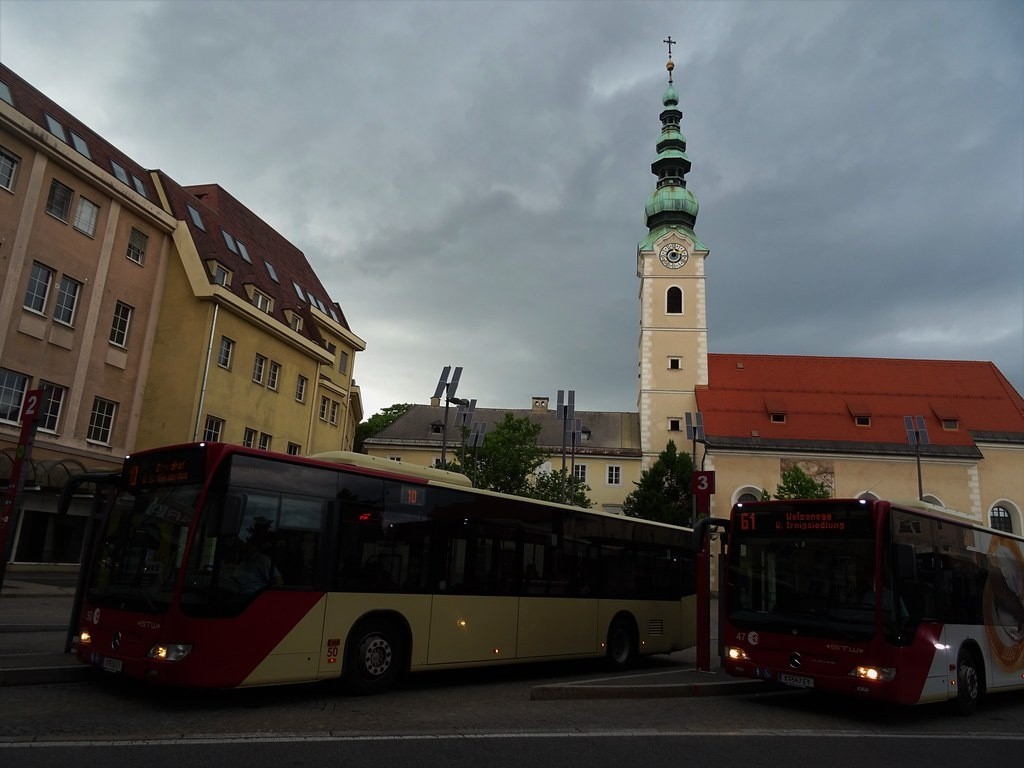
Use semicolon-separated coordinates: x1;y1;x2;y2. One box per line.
659;243;688;269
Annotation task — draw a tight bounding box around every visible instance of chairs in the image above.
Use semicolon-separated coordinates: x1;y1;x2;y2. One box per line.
496;574;527;594
238;521;306;586
888;581;926;627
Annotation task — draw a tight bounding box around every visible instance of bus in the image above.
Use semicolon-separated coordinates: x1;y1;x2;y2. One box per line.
55;441;697;693
693;497;1024;716
